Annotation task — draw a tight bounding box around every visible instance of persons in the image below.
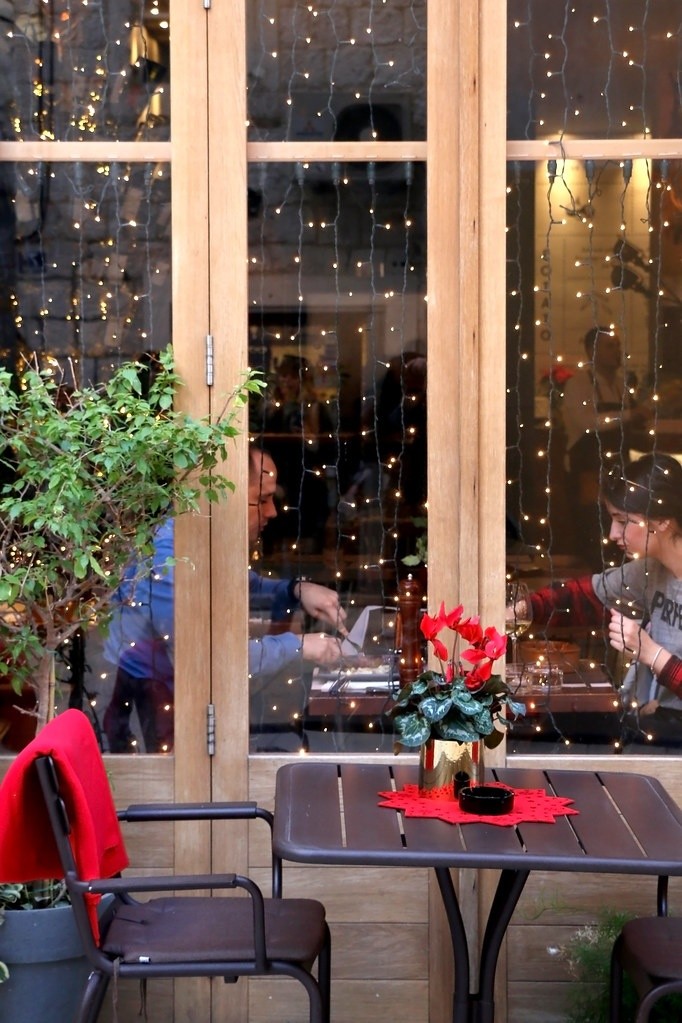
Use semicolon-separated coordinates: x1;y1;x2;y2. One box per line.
505;450;682;748
124;350;428;606
561;324;648;574
94;446;350;754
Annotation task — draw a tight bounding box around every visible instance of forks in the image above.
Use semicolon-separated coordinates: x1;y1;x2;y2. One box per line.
343;634;365;658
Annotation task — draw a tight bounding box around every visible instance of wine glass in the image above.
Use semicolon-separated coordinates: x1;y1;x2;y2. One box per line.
506;581;533;679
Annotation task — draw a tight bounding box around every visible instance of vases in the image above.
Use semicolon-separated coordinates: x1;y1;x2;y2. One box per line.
417;729;486;800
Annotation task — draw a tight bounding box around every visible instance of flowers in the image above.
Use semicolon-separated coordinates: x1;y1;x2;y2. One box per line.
386;602;525;749
541;366;576;385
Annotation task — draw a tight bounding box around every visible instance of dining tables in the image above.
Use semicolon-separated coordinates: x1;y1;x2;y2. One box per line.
309;644;619;753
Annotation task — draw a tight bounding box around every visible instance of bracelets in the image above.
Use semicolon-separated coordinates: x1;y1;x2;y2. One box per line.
649;647;665;674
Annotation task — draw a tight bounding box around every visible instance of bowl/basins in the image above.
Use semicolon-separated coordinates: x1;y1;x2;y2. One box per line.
517;639;582;673
528;667;564;690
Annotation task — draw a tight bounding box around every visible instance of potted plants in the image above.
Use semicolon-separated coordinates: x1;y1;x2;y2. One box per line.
1;337;268;1021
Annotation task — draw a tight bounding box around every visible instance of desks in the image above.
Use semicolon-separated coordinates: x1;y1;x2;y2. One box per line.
274;763;682;1022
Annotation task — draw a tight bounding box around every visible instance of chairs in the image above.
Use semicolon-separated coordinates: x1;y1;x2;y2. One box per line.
609;916;681;1023
2;706;333;1023
